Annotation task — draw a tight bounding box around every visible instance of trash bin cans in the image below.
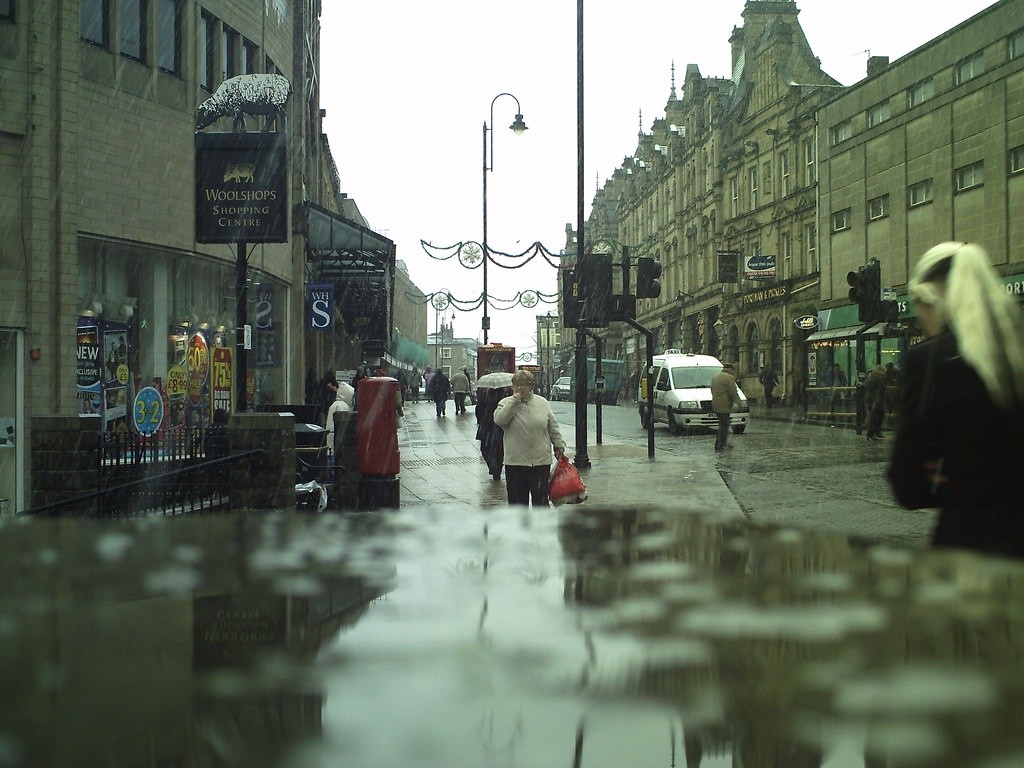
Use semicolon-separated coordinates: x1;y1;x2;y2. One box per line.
357;377;400;475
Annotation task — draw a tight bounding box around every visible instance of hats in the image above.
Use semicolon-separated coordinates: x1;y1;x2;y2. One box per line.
723;363;734;369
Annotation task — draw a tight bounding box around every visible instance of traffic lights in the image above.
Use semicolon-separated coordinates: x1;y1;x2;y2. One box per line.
650;261;661;298
848;270;875;302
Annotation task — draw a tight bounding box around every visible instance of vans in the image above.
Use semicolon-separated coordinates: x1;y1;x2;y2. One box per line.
637;349;749;434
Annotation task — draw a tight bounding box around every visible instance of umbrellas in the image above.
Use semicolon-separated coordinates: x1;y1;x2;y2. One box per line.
476;373;514;389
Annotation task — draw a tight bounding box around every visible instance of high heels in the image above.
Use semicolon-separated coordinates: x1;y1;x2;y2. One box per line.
866;434;878;441
876;433;884;438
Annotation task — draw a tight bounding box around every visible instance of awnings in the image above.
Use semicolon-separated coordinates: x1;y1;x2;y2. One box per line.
805;325;884;342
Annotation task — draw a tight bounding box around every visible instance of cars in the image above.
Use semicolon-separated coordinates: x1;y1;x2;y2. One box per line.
408;376;432;400
550;377;572;401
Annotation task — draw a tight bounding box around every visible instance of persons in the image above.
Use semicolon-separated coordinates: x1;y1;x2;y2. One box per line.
393;365;435;404
711;362;742;451
856;362;902;442
475;387;514;481
493;370;565;507
887;242;1024;560
397;385;404;432
326;381;356;481
829;363;847;412
428;368;451;418
759;363;779;411
322;381;338;410
449;365;471;416
351;358;398;388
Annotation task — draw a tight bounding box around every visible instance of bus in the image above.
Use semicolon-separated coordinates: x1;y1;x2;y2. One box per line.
567;356;626;405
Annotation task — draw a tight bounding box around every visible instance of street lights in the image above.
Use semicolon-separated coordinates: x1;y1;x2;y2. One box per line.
536;311;553;401
482;90;528;344
435;287;456;371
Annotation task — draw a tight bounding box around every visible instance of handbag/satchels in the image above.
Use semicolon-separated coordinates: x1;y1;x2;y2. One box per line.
464;395;472;406
548;455;588;505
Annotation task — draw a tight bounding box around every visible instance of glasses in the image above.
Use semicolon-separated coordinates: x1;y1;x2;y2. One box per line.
513;384;528;388
